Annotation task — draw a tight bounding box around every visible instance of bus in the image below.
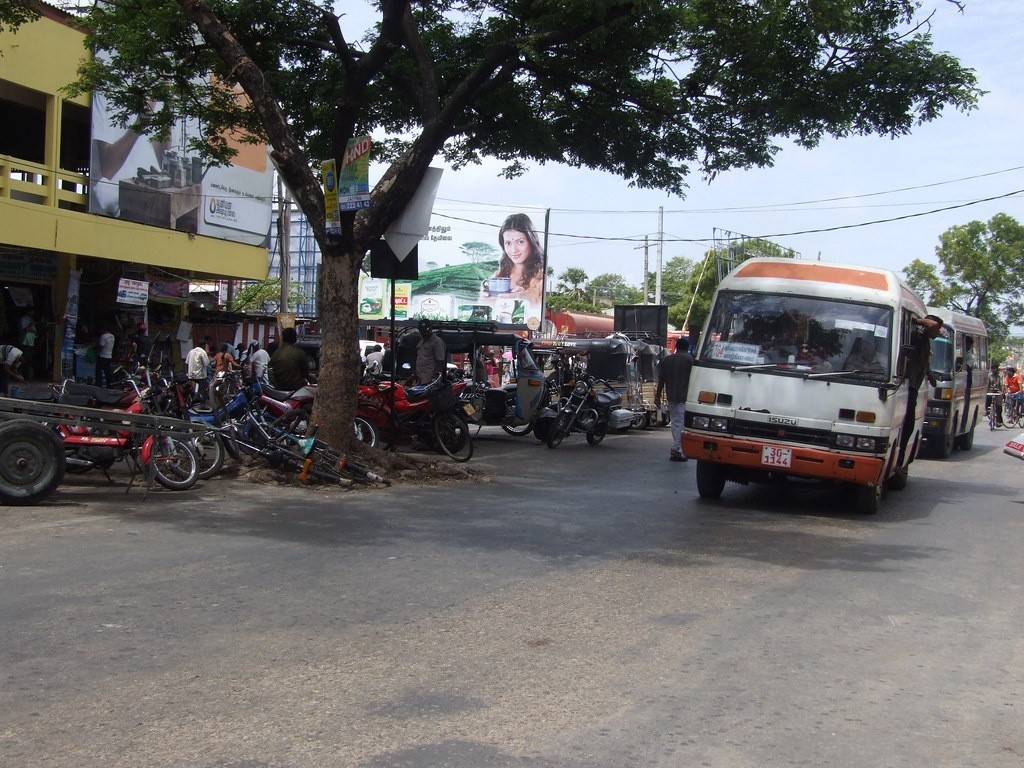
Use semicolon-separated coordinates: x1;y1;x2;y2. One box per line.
667;331;733;357
925;304;989;459
670;256;929;514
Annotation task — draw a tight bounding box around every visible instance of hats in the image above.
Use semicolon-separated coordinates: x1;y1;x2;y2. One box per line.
250;340;259;347
419;319;430;328
235;343;244;350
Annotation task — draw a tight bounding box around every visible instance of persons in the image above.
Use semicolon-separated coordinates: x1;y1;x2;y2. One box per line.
0;345;30;397
687;325;701;356
846;334;887;374
895;314;943;480
185;335;271;386
654;338;693;461
986;365;1024;431
482;213;544;304
94;323;115;388
382;343;394;373
366;345;383;374
405;319;445;386
18;306;36;356
965;336;973;373
572;350;591;370
269;328;309;391
547;362;576;398
502;364;511;384
93;78;173;218
128;322;152;361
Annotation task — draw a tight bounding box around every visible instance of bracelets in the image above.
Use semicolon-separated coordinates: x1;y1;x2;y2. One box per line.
411;374;415;377
655;394;662;397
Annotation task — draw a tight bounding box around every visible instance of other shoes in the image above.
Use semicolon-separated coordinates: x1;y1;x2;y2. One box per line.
984;411;990;415
1007;418;1014;424
199;406;210;410
895;465;907;479
996;422;1002;427
670;447;688;461
416;442;430;451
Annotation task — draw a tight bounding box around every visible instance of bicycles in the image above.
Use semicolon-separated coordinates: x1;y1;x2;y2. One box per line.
988;386;1024;431
225;402;392;489
358;371;475;462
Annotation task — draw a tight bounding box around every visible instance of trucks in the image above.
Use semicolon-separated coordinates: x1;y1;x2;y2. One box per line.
531;311;615;337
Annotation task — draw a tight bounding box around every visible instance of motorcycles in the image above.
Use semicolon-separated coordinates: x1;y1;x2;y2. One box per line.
187;364;283;467
41;333;223;490
256;378;318;436
357;381;469;443
546;361;622;450
459;377;533;436
365;319;672;430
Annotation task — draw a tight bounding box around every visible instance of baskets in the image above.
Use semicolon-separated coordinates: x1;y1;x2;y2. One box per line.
432;386;457;412
